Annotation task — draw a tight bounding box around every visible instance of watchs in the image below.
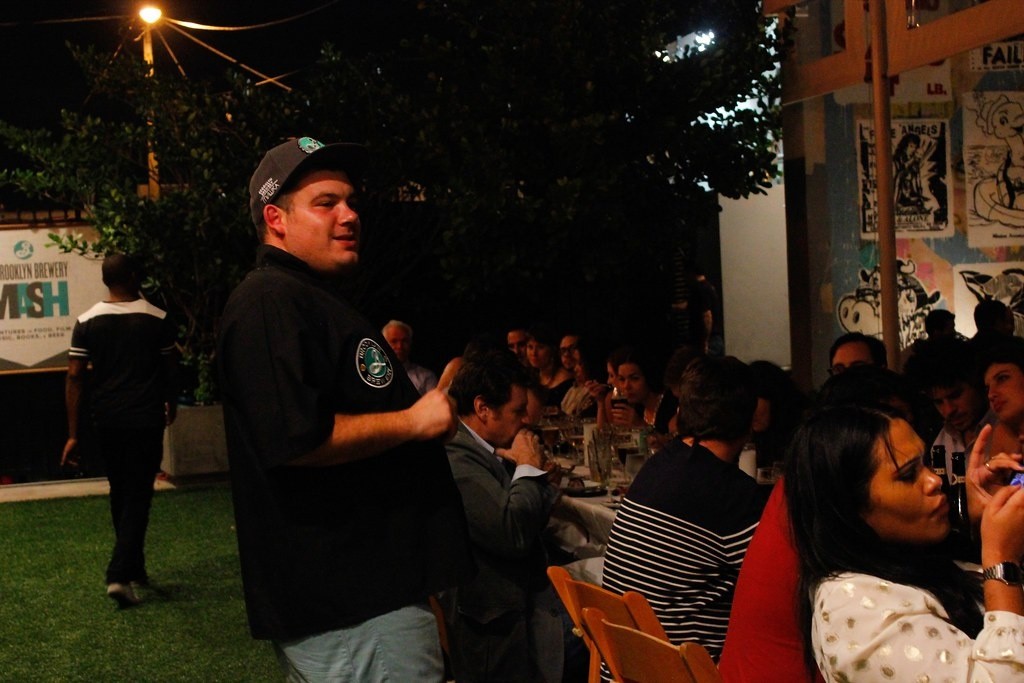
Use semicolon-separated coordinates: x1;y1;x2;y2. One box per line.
982;562;1023;585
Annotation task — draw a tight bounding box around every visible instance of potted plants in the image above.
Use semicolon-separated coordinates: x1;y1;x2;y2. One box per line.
0;39;478;479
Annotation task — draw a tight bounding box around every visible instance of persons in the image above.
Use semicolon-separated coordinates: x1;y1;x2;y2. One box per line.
221;139;454;683
60;255;181;607
689;273;719;355
803;406;1024;683
441;361;603;653
380;301;1024;490
598;355;794;683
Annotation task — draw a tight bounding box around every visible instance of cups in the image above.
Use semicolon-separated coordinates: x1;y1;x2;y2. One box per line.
738;443;758;480
531;405;639;486
625;453;651;480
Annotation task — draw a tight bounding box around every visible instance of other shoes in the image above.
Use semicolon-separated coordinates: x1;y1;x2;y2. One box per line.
132;574;147;586
108;582;138;610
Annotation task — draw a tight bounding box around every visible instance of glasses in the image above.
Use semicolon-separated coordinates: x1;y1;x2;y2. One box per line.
508;339;528;348
826;360;868;376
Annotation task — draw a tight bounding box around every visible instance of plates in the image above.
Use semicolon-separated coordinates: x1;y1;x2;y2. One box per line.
564;489;609;497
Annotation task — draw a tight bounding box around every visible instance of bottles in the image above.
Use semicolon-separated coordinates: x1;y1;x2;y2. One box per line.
931;445;948;497
949;452;972;556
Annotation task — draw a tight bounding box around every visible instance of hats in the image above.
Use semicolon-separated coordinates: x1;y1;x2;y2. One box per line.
249;137;358;233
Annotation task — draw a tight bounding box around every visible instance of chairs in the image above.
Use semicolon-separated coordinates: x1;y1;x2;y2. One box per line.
580;606;725;683
546;566;670;683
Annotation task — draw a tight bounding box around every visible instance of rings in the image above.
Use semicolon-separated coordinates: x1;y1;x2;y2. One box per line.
991;457;995;459
985;463;997;474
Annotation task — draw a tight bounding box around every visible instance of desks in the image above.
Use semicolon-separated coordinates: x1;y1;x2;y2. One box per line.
548;457;986;612
